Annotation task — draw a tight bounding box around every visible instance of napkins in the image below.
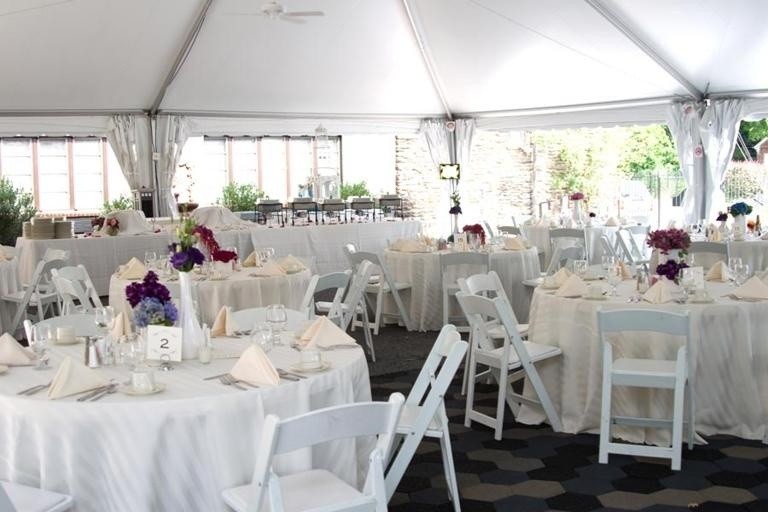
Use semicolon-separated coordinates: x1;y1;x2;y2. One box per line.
111;313;133;338
46;355;105;401
210;305;239;337
230;343;281;387
300;313;358;350
0;333;36;367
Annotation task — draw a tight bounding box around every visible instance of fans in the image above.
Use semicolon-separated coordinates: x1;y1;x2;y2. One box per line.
223;0;324;23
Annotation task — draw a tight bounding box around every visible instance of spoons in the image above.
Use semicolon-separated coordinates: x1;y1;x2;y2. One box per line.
276;367;308;380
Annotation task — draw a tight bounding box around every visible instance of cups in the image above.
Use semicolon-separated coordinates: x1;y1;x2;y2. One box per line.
299;349;321;369
132;366;154;391
257;247;273;269
56;327;76;344
208;269;226;280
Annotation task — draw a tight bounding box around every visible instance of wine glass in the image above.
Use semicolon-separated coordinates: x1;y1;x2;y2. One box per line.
95;305;115;361
29;322;50;370
120;333;144;385
727;256;749;287
250;305;287;355
574;255;622;297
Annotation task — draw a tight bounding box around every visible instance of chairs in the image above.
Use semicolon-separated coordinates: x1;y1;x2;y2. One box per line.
457;270;531;395
384;323;470;511
315;260;376;362
223;391;406;511
593;307;696;468
1;195;767;307
453;289;563;441
3;256;65;337
1;480;72;511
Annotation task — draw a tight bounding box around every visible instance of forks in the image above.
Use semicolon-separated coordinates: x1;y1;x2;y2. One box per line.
219;374;259;391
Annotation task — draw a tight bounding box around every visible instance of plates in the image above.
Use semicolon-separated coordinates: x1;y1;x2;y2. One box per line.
290;360;330;373
119;383;165;396
22;216;74;240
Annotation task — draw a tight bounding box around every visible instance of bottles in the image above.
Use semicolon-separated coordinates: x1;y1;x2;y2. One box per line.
756;215;760;236
636;264;650;294
84;334;98;369
